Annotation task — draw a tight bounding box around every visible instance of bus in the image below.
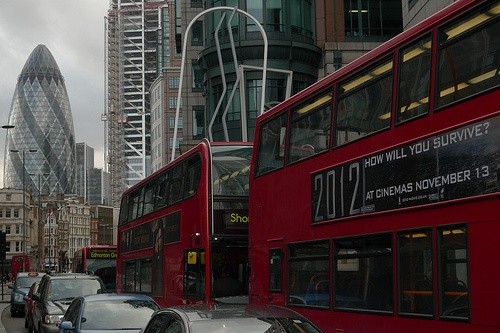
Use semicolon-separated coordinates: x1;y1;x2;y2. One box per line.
246;0;500;333
116;137;317;309
11;254;39;280
71;245;118;293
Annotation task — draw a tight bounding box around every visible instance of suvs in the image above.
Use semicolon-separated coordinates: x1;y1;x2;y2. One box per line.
30;270;110;333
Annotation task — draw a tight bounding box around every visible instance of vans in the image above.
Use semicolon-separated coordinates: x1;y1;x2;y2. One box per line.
8;272;47;317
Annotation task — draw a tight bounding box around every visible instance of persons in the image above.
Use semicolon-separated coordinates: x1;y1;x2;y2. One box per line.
2;273;10;284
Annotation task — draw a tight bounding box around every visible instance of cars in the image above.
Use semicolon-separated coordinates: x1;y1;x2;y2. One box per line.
58;292;164;333
23;280;42;333
139;303;324;333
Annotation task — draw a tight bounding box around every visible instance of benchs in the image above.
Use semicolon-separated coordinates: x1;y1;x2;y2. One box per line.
290;271;365;305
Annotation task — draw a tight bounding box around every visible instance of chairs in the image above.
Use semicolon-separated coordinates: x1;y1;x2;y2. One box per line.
305;273;470;317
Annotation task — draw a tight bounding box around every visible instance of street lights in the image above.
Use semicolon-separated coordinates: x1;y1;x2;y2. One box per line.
9;148;38;271
29;173;76;274
